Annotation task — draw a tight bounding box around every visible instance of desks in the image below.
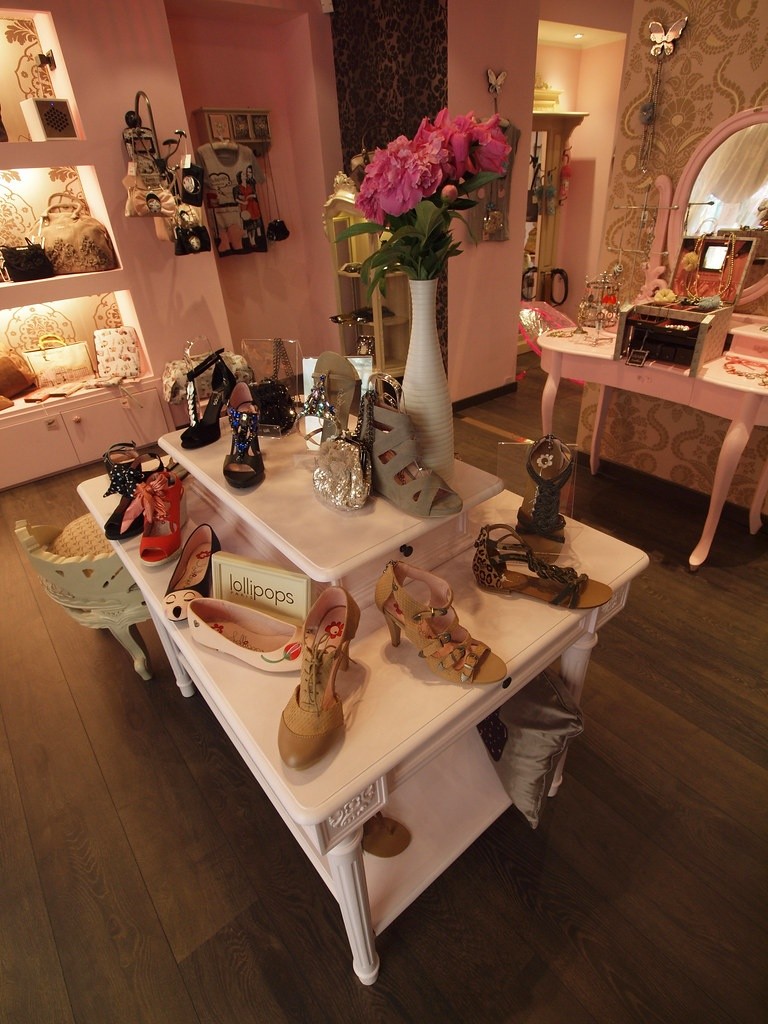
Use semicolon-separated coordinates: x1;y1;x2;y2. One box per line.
537;327;768;572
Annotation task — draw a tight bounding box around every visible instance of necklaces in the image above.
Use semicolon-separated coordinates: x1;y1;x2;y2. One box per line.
546;232;768;386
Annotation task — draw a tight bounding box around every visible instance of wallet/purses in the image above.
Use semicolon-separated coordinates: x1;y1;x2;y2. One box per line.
49;383;85;400
85;377;144;409
24;386;58;417
0;395;14;411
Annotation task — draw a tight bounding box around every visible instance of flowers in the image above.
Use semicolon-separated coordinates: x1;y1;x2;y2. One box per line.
334;109;514;306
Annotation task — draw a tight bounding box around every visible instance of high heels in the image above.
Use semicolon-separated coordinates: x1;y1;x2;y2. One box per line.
375;559;508;686
278;586;361;771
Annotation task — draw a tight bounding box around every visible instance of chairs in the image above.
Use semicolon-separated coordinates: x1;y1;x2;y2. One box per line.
14;511;152;680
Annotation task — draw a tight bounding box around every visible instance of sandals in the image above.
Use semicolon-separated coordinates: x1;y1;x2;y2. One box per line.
223;381;266;489
472;524;613;609
515;434;574;564
181;348;237;449
120;471;185;567
102;440;140;481
103;453;165;540
359;398;463;519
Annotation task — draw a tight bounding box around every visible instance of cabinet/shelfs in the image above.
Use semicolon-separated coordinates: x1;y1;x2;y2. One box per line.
0;139;169;489
76;395;649;986
332;211;412;378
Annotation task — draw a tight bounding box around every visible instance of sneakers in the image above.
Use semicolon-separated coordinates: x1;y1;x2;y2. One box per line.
187;598;303;674
163;523;221;622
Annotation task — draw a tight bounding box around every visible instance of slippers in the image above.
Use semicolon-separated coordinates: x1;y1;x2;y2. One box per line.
295;351;356;449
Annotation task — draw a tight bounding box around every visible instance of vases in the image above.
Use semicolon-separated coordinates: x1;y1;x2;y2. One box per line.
397;278;455;481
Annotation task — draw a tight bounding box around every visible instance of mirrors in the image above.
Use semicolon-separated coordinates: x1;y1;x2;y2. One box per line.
668;106;768;307
518;130;548;333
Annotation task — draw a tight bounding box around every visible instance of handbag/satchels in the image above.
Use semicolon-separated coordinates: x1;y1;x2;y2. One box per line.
173;225;211;256
182;138;204;207
311;390;377;512
121;127;177;218
93;326;141;379
267;219;289;242
246;338;298;434
0;237;56;283
21;334;97;390
148;194;203;243
0;348;37;399
23;192;117;275
